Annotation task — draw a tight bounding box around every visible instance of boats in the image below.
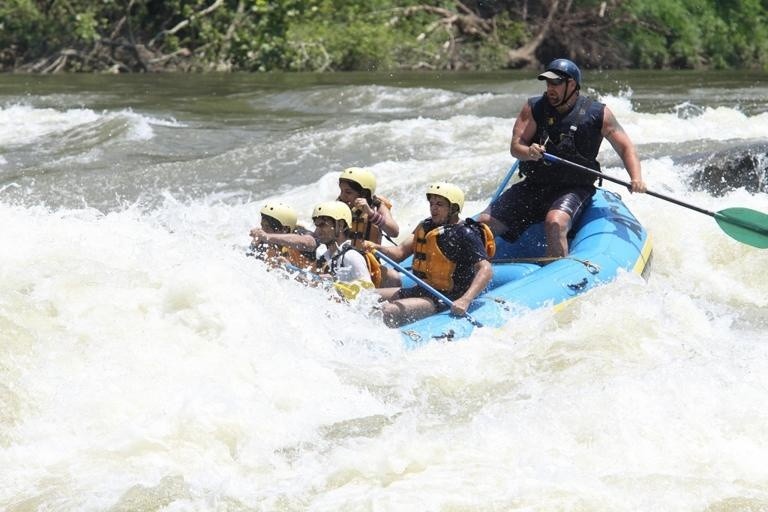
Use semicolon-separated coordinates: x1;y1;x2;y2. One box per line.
381;187;654;352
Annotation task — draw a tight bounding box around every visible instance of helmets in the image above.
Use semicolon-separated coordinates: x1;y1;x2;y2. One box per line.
339;166;377;201
260;201;298;233
425;182;466;214
539;58;581;90
312;200;353;229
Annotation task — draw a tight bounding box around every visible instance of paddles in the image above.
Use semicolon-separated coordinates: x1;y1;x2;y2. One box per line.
248;248;359;301
540;150;767;248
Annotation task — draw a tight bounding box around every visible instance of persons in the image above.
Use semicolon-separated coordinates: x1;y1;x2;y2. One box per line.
333;164;400;263
248;201;319;270
272;200;374;301
361;180;493;328
475;59;647;265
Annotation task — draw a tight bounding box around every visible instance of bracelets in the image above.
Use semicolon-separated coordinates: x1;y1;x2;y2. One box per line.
368;211;386;228
